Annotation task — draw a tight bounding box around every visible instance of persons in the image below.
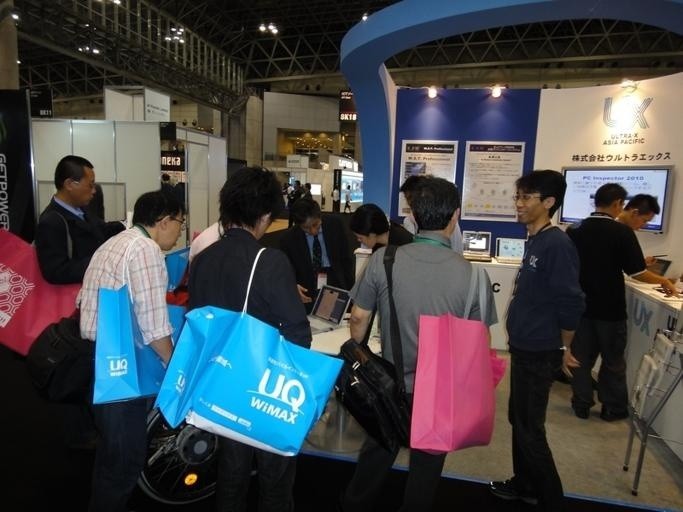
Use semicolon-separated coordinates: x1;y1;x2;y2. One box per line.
339;175;500;510
488;168;587;512
564;181;682;422
39;153;415;512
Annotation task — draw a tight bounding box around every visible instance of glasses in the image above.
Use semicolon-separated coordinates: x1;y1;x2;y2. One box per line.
513;195;541;200
157;215;186;230
73;181;96;188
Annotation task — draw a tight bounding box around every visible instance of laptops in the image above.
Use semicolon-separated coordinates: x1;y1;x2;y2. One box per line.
462;230;492;262
495;237;527;263
305;284;351;336
623;255;672;285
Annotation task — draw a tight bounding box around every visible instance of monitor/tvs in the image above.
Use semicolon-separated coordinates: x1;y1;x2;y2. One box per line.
557;165;675;237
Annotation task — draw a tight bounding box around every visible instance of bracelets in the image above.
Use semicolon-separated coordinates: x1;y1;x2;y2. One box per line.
559;345;571;352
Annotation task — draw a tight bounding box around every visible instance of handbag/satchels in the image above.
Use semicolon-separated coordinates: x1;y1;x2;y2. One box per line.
333;338;411;448
93;285;187;405
152;306;346;457
410;313;507;456
0;227;83;355
165;247;190;292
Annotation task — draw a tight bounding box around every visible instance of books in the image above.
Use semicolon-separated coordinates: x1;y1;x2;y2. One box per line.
461;250;492;262
495;255;522;265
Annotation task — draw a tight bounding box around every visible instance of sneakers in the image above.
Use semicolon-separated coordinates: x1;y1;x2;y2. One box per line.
490;475;539;505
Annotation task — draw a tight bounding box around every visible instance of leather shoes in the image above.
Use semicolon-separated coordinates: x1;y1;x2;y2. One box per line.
572;404;590;419
600;407;628;421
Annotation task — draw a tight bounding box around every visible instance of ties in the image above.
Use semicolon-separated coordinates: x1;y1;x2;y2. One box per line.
313;235;322;272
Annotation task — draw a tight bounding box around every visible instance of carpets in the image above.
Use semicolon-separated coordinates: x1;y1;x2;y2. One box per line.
0;343;678;511
